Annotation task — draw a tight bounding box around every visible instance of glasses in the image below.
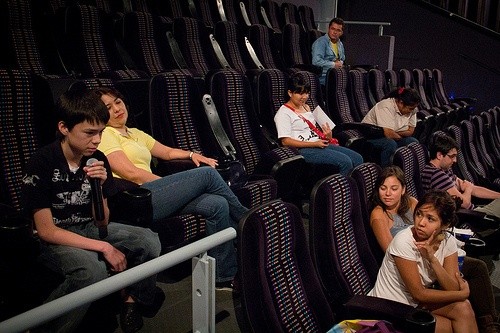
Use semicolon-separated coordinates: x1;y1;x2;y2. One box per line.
445;153;458;159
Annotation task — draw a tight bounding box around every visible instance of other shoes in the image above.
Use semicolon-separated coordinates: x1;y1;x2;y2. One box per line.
215;279;235;292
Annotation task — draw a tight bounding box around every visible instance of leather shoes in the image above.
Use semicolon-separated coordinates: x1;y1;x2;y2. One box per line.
120;301;143;333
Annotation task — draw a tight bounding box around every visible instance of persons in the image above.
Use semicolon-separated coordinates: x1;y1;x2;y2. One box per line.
312;18;346;86
367;165;499;333
361;86;422;168
96;86;249;291
367;188;477;333
273;74;363;176
420;131;500;259
24;86;162;333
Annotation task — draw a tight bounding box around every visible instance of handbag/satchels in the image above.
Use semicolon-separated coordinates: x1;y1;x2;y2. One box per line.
323;137;338;146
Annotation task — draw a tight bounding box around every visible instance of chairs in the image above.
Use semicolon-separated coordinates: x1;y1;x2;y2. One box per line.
0;0;326;75
79;78;232;250
0;65;153;230
208;67;260;178
258;66;500;238
232;198;391;333
149;72;200;187
309;174;437;333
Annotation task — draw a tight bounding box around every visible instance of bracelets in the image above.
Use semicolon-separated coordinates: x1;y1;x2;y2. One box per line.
189;151;195;159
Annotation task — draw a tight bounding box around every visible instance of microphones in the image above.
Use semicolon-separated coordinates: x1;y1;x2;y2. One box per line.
86;158;105;222
